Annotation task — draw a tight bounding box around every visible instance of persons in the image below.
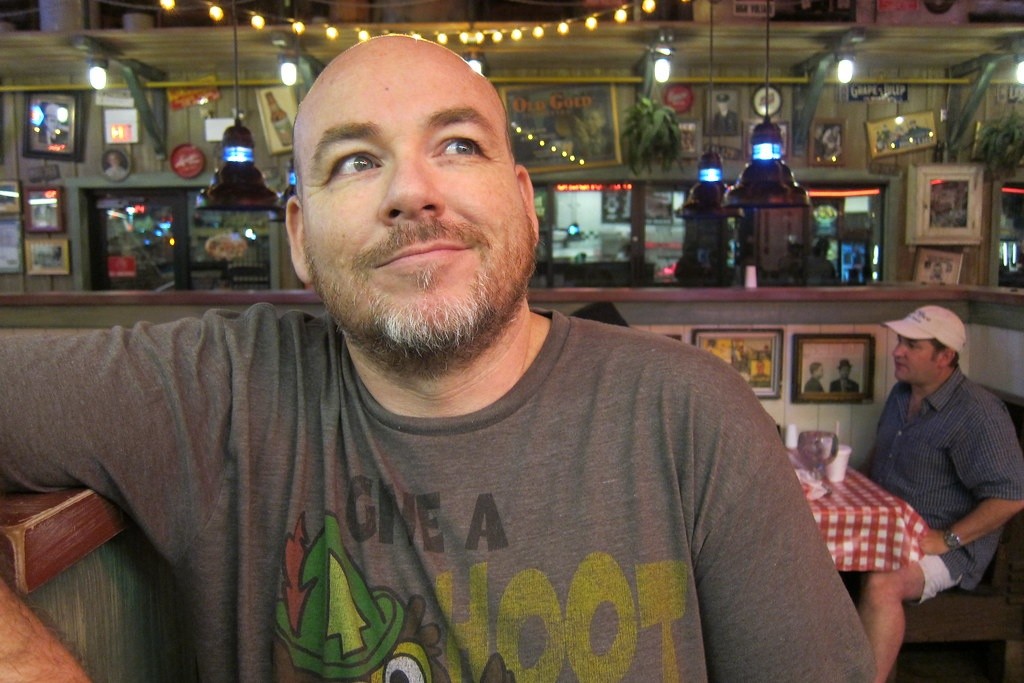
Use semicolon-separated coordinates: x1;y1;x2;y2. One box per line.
778;243;806;285
0;34;877;683
876;119;932;152
829;359;859;392
804;362;825;392
854;305;1024;683
572;100;613;158
40;104;69;145
803;240;837;286
814;122;842;162
712;94;737;132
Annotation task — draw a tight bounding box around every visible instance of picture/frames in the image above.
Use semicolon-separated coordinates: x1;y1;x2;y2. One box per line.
701;86;742;138
866;110;938;159
665;121;698;157
25;184;65;232
0;179;22;219
749;122;788;161
100;146;132;184
0;219;24;275
25;236;72;277
694;326;780;397
912;247;969;284
905;162;985;245
807;120;849;166
496;80;620;172
790;334;877;403
751;84;782;118
24;92;88;162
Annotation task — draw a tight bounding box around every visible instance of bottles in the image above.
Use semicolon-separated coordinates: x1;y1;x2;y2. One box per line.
744;264;758;287
785;423;798;450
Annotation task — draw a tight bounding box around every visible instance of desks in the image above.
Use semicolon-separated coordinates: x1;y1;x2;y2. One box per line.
784;444;928;572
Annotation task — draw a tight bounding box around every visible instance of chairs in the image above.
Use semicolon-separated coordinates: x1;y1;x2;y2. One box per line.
845;384;1023;683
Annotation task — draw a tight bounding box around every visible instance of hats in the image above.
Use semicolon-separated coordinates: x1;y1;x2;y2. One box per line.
882;304;966;353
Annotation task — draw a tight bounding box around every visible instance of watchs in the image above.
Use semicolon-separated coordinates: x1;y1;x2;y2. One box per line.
944;527;961;550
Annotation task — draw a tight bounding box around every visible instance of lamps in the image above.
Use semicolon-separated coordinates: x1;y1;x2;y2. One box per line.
675;0;747;222
191;0;279;212
720;0;815;209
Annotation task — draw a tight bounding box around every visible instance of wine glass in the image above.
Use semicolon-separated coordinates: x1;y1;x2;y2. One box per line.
797;430;837;495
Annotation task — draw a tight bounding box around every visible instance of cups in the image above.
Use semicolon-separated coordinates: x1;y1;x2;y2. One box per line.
826;444;851;484
